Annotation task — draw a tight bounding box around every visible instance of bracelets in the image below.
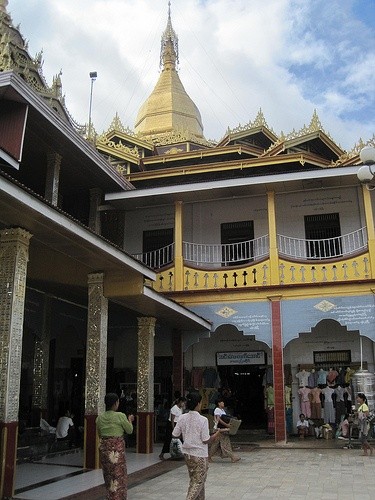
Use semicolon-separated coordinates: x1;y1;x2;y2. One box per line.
214;434;216;436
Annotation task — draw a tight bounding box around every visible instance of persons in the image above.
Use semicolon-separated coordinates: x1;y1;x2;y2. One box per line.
262;367;356;434
159;397;187;461
56;410;75;449
172;392;220;500
296;414;310;439
206;398;241;463
96;393;135;500
341;393;375;456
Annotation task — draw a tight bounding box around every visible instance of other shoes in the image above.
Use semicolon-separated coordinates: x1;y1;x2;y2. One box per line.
361;449;368;455
231;456;241;463
158;453;165;461
369;448;375;457
